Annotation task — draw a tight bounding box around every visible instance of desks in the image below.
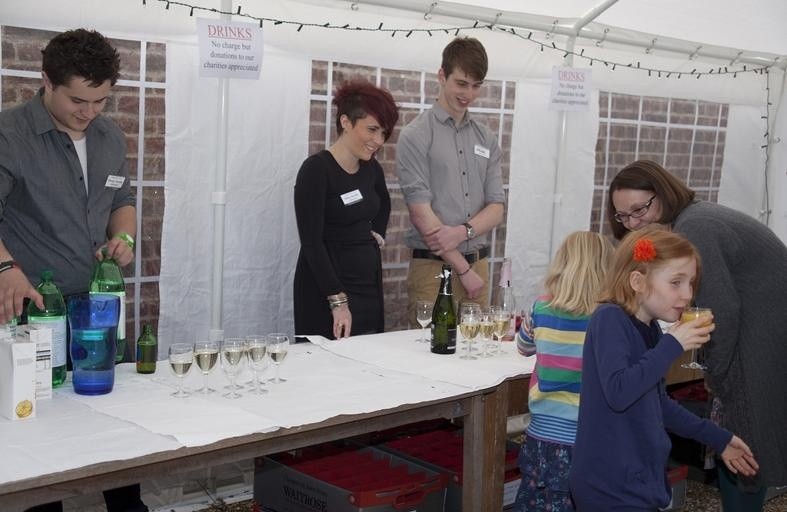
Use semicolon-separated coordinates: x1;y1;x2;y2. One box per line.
340;329;715;512
0;345;486;511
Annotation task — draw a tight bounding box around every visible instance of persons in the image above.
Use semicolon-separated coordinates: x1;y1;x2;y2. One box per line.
293;77;400;343
606;161;787;511
569;223;760;512
398;35;505;329
0;28;137;368
515;232;615;512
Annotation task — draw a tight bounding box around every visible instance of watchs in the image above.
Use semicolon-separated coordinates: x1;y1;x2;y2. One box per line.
113;233;134;249
462;223;475;241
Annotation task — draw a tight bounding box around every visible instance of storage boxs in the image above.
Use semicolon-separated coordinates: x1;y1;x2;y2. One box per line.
246;440;448;511
354;423;526;511
669;377;715;418
660;454;694;510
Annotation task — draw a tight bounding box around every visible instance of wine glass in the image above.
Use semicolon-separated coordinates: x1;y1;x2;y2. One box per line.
168;332;291;402
455;301;511;360
679;305;713;372
414;299;436;344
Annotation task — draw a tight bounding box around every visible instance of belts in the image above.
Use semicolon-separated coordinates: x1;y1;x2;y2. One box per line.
413;247;490;266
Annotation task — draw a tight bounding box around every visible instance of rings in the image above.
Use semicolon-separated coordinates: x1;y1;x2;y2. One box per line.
337;320;343;327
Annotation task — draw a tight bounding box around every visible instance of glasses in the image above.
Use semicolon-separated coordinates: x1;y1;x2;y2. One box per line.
614;194;657;223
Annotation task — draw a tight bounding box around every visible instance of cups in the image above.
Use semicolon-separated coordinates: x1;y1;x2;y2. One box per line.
66;293;122;396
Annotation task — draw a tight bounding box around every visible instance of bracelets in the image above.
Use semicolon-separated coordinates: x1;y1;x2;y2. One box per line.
328;295;350;310
0;259;19;273
457;265;473;277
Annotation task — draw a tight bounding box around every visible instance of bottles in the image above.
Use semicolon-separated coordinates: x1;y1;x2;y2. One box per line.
91;244;127;362
494;257;516;342
430;262;458;355
6;315;18;343
28;268;68;391
136;323;159;374
